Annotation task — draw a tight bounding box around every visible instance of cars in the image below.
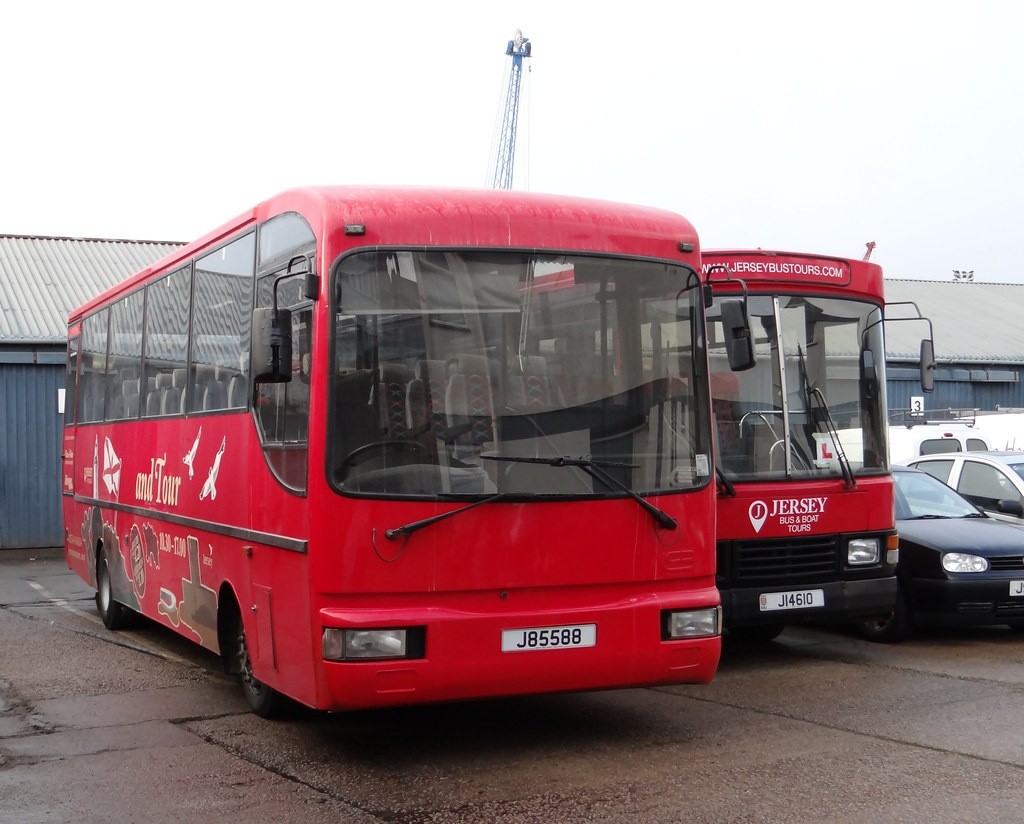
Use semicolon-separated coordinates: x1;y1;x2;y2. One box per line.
846;461;1024;643
900;450;1024;534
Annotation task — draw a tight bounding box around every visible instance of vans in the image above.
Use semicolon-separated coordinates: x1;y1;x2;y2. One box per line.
819;409;994;473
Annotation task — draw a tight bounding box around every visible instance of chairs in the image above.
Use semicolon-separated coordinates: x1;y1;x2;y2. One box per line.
84;354;550;465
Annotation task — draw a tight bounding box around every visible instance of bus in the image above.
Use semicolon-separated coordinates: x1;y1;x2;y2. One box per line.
526;252;938;658
61;183;754;719
